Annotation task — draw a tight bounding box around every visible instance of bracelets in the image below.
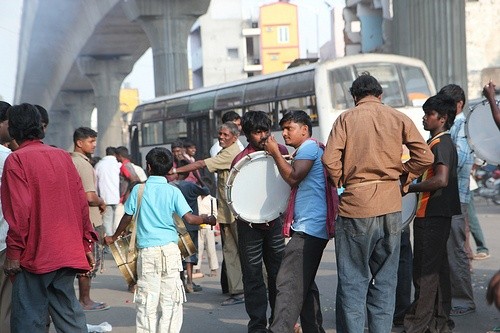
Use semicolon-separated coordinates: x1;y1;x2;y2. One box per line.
173;168;176;173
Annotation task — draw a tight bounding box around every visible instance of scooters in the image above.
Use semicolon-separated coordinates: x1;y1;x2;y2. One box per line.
470;158;500;207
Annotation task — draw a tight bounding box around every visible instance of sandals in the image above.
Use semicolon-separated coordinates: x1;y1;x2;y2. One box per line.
220;296;244;306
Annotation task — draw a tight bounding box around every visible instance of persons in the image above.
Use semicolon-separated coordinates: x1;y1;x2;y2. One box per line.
102;146;209;333
0;71;500;333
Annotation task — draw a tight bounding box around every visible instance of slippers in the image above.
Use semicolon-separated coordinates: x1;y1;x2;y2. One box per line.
82;302;110;311
449;305;475;316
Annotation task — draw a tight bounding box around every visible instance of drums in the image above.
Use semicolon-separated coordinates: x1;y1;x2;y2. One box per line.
226;150;292;223
401;177;418;228
464;90;500;167
109;212;196;283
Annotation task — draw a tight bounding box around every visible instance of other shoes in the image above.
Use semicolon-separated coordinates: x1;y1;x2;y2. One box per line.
477;247;489;257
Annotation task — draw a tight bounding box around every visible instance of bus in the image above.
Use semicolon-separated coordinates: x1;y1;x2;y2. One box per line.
122;53;433;199
